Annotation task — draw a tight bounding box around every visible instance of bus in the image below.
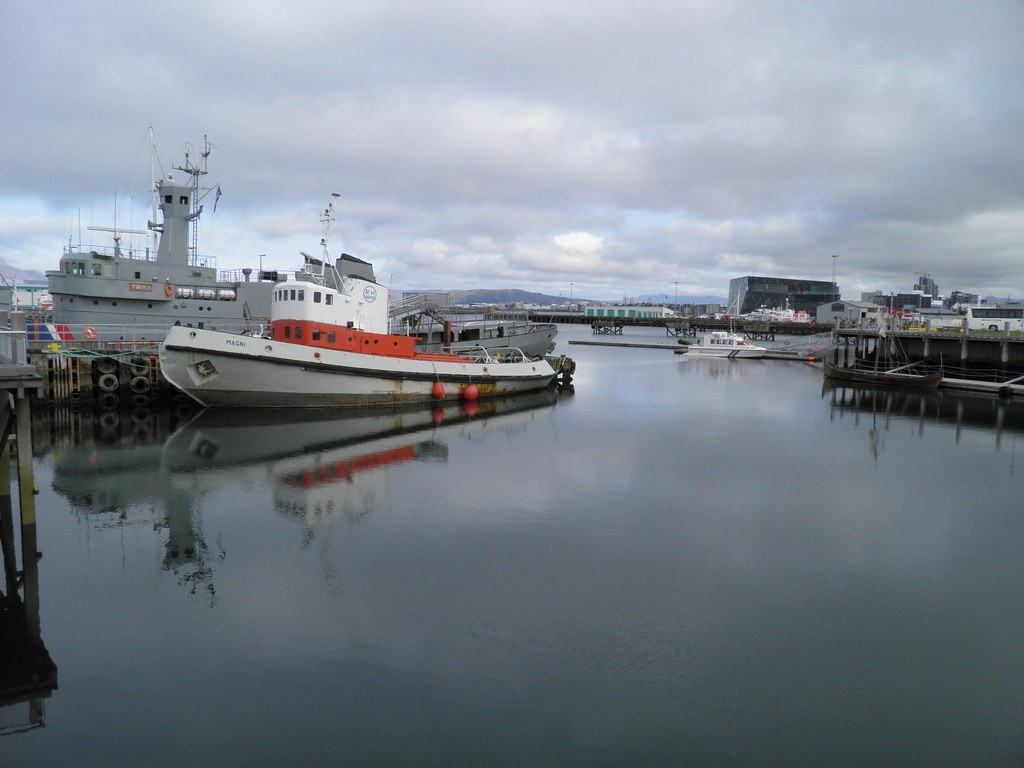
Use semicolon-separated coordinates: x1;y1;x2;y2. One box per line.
966;303;1024;332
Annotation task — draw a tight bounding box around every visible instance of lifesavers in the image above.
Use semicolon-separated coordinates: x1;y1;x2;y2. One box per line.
97;358;119;374
88;452;103;465
98;374;119;391
164;285;173;297
84;327;97;339
129;357;151;376
98;401;120;441
98;392;120;401
130;394;150;400
130;376;150;393
130;400;151;442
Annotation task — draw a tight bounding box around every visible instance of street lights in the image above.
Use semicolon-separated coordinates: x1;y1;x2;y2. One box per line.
570;283;576;306
674;282;679;306
832;254;840;303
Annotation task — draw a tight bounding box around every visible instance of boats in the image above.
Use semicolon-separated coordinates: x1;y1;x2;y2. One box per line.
822;305;946;387
157;395;572;571
41;122;561;402
684;329;764;360
153;248;576;405
50;386;571;607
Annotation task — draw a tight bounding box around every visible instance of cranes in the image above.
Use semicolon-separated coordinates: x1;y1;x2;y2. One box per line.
913;270;932;279
